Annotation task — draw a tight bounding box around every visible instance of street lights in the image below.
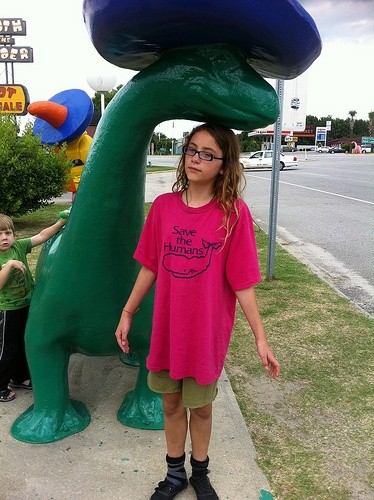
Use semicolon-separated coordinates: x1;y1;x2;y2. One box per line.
87;70;116;114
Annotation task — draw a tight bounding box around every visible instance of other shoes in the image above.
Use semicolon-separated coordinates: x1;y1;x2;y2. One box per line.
188;476;219;500
149;480;189;500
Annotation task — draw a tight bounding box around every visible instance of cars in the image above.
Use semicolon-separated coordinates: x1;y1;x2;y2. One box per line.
232;149;301;170
329;145;349;154
282;146;299;152
316;146;332;153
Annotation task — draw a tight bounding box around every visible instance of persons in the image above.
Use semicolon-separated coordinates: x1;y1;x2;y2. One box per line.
115;123;281;500
0;210;71;402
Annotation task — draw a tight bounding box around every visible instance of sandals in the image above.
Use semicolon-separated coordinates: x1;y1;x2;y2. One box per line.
9;379;33;390
0;389;16;402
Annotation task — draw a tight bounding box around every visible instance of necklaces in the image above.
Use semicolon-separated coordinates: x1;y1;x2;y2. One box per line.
185;190;216;207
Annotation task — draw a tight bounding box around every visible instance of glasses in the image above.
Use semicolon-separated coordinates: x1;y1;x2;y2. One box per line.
183;146;224;161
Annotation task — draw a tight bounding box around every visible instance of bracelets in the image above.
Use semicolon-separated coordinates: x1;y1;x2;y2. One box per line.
121;308;139;315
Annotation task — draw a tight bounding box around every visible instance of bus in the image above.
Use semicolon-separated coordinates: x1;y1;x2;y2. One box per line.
361;143;372;154
